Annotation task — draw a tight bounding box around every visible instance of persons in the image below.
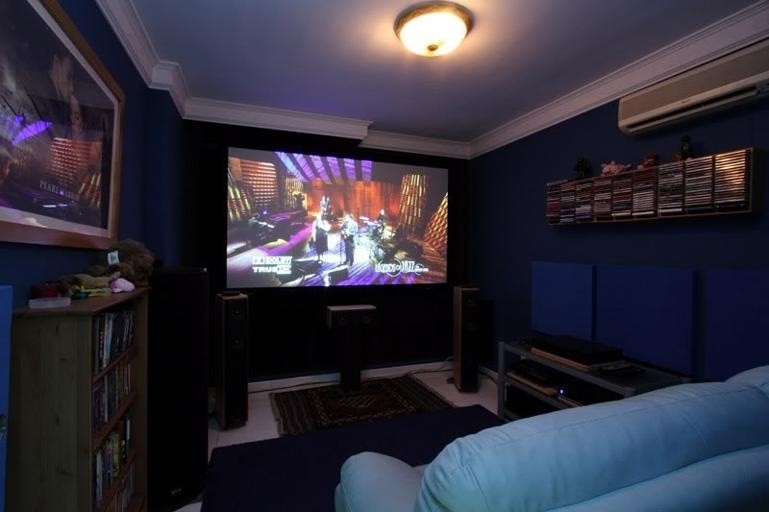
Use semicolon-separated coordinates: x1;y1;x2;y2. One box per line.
2;49;100;215
311;212;331;265
340;213;358;264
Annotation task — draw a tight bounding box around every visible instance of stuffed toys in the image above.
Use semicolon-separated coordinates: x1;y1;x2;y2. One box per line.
71;239;154;293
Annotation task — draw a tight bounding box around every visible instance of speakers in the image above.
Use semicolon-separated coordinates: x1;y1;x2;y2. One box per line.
452;286;481;392
215;294;248;429
326;304;377;330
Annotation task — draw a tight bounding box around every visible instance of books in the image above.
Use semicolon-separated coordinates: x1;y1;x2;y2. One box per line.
94;307;134;512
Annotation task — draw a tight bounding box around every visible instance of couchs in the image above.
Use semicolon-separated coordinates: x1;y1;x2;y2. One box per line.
332;362;769;510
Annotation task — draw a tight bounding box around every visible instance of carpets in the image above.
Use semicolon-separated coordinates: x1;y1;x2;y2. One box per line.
200;369;510;511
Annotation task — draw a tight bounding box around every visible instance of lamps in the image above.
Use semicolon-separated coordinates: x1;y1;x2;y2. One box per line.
392;1;476;59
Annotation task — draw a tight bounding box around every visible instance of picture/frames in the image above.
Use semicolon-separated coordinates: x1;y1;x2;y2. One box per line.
0;0;126;256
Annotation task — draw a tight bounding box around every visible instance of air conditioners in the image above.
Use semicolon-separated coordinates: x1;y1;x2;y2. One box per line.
615;38;768;142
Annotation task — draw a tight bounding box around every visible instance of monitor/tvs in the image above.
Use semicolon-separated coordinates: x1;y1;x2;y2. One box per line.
225;145;449;290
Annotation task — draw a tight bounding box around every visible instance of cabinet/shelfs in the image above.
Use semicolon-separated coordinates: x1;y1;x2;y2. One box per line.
7;282;152;511
495;333;695;420
